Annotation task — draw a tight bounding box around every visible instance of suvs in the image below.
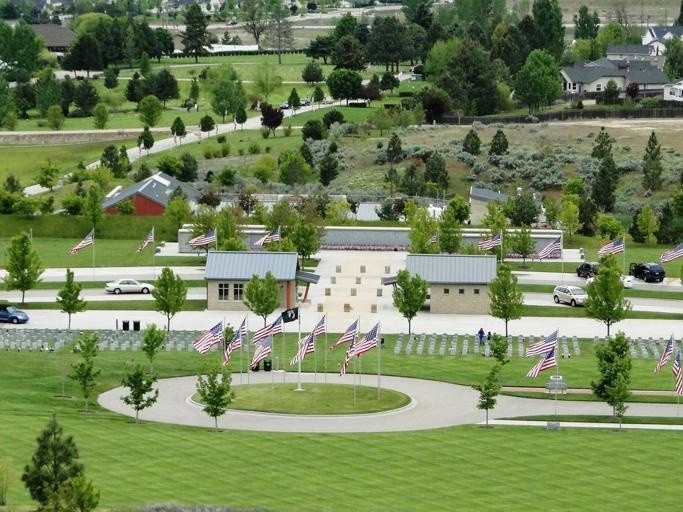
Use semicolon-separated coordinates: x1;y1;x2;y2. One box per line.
628;261;666;283
575;261;600;276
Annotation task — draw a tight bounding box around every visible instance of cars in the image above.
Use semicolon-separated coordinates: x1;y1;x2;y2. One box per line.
0;303;28;324
299;98;309;107
586;273;634;288
105;278;155;294
280;101;288;109
321;97;333;105
553;285;588;306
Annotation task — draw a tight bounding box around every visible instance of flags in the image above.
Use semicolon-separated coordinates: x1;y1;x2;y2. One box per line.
653;336;683;397
136;231;152;253
597;236;624;259
477;232;501;251
525;330;559;378
538;236;561;260
424;232;437;246
69;231;93;255
253;227;279;246
660;242;683;262
194;306;380;376
188;230;216;249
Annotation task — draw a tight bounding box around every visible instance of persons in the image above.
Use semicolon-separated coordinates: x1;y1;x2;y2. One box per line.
478;328;485;345
381;335;384;349
487;331;491;340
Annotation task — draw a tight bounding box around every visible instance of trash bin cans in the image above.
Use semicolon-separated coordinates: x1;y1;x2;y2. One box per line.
134;321;140;331
123;322;129;331
252;363;259;371
264;359;272;371
